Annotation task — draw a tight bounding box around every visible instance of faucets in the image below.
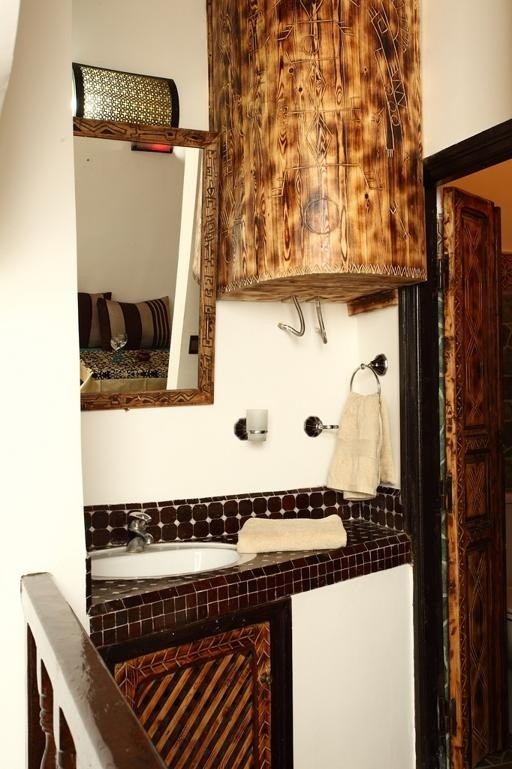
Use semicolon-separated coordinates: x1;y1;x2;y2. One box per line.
120;510;156;554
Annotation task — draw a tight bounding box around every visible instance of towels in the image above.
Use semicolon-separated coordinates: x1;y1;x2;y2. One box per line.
323;391;396;502
236;513;348;554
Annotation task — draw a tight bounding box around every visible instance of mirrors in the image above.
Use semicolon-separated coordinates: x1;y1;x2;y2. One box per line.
73;116;221;412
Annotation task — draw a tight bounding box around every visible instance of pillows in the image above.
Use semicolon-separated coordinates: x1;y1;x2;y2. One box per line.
78;291;172;353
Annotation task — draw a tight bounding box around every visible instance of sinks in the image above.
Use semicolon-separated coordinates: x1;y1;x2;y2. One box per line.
86;538;259;582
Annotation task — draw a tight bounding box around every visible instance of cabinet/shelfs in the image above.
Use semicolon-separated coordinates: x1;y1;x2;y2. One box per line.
95;596;292;768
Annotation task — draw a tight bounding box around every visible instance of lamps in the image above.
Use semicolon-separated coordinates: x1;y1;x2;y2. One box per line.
234;408;270;444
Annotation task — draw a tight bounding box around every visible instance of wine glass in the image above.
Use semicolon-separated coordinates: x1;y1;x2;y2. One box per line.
110;333;128;358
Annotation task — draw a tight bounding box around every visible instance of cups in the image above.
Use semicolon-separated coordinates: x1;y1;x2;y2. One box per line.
247;409;269;442
